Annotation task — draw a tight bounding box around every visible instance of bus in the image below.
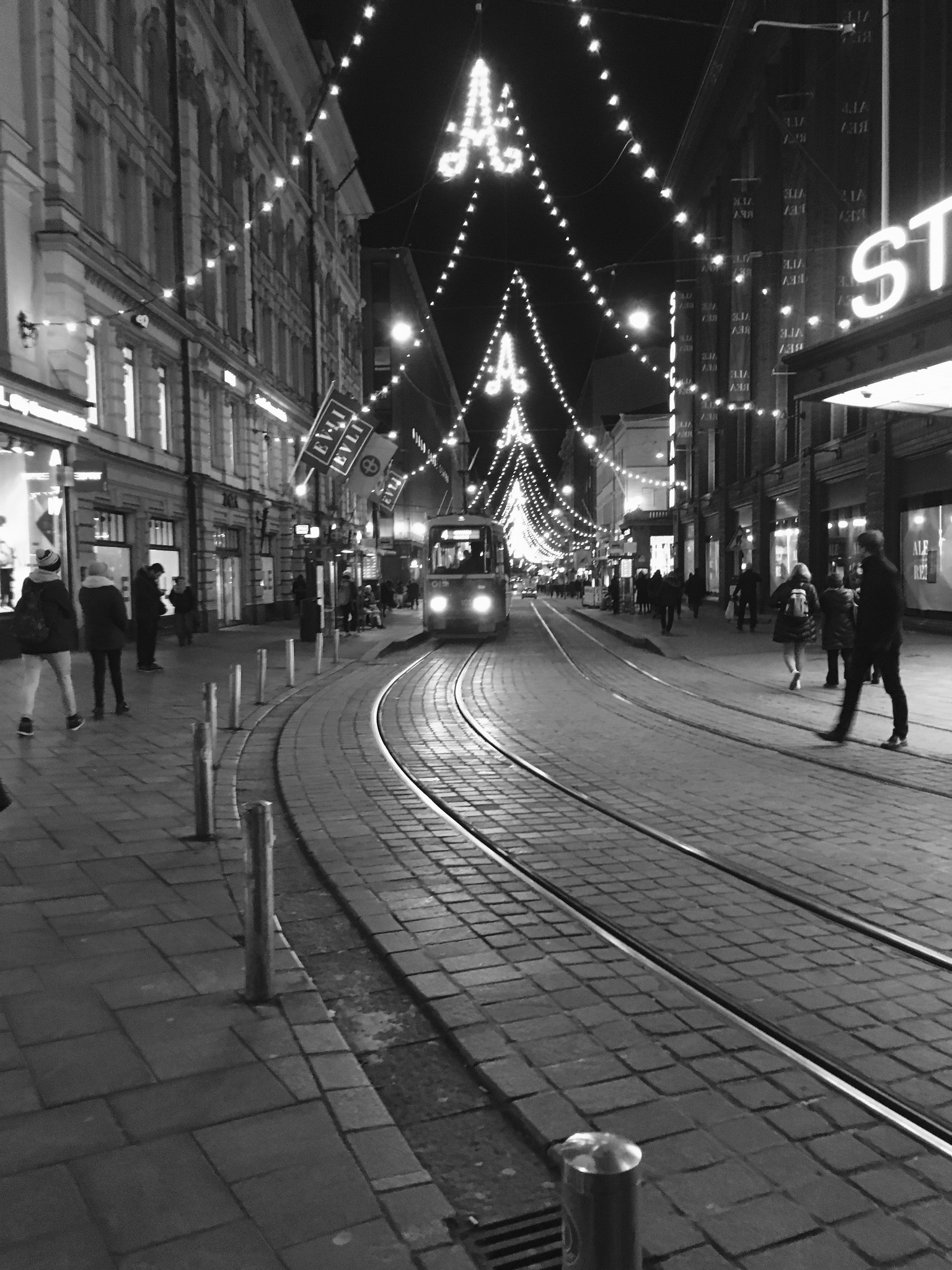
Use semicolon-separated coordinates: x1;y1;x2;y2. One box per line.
422;469;510;640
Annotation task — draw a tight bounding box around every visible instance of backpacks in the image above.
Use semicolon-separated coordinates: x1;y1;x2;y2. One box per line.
783;580;810;622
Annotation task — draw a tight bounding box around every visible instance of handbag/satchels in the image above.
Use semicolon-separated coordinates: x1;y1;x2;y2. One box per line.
724;600;734;620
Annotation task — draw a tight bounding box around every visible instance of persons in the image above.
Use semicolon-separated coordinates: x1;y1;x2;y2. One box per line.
600;574;620;614
292;571;419;632
79;562;129;720
536;575;592;599
731;563;762;633
770;563;821;690
169;576;195;647
634;568;707;637
132;563;166;671
817;529;908;749
14;549;85;736
0;515;15;607
458;550;471;569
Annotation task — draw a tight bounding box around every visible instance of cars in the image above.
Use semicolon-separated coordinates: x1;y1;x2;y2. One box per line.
521;588;537;599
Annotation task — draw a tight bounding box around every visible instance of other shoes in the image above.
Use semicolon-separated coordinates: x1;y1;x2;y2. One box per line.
736;625;742;629
114;703;130;715
138;664;155;672
17;720;34;735
750;623;756;628
66;716;85;731
872;677;879;684
795;680;801;690
823;682;837;689
152;663;165;671
92;707;103;720
789;670;800;690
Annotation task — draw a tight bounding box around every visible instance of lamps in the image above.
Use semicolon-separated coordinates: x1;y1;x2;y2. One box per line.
0;438;37;456
256;500;272;554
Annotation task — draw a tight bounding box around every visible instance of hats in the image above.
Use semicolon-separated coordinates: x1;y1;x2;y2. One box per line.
35;548;61;571
88;563;108;577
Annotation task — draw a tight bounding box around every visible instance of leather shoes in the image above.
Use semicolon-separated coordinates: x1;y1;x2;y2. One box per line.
881;736;907;748
818;730;844;743
